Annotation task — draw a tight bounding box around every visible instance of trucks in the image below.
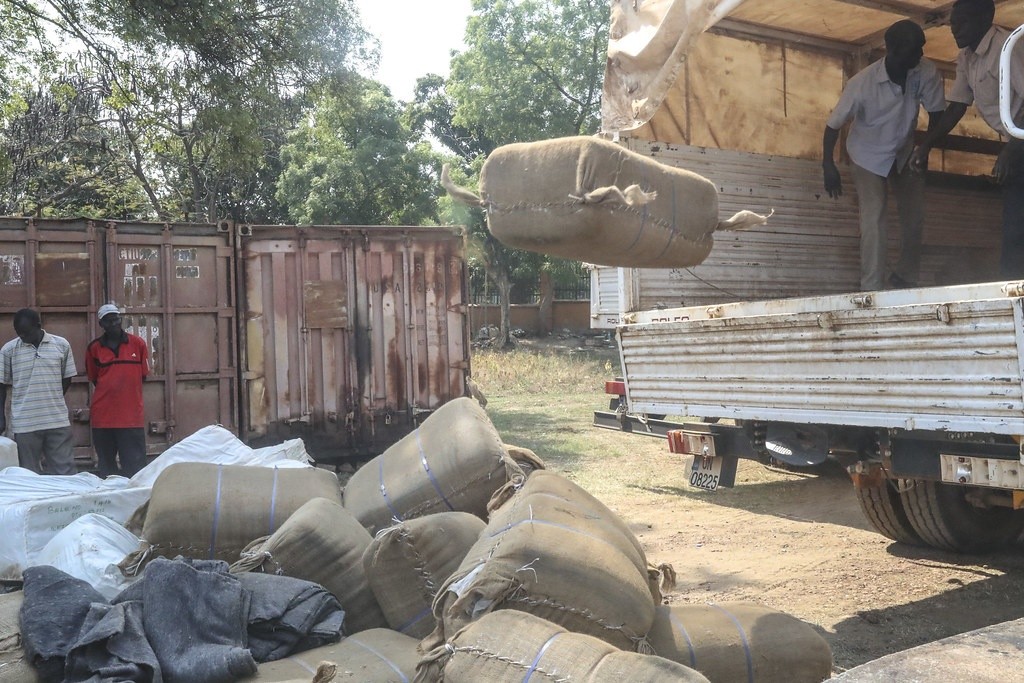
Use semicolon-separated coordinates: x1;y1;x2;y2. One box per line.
589;0;1024;550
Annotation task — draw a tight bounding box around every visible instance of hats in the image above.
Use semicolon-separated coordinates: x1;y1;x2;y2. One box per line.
97;304;121;321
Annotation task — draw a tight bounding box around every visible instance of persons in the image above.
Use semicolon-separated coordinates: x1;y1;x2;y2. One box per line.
823;20;947;292
908;0;1024;282
0;308;77;475
85;304;150;479
